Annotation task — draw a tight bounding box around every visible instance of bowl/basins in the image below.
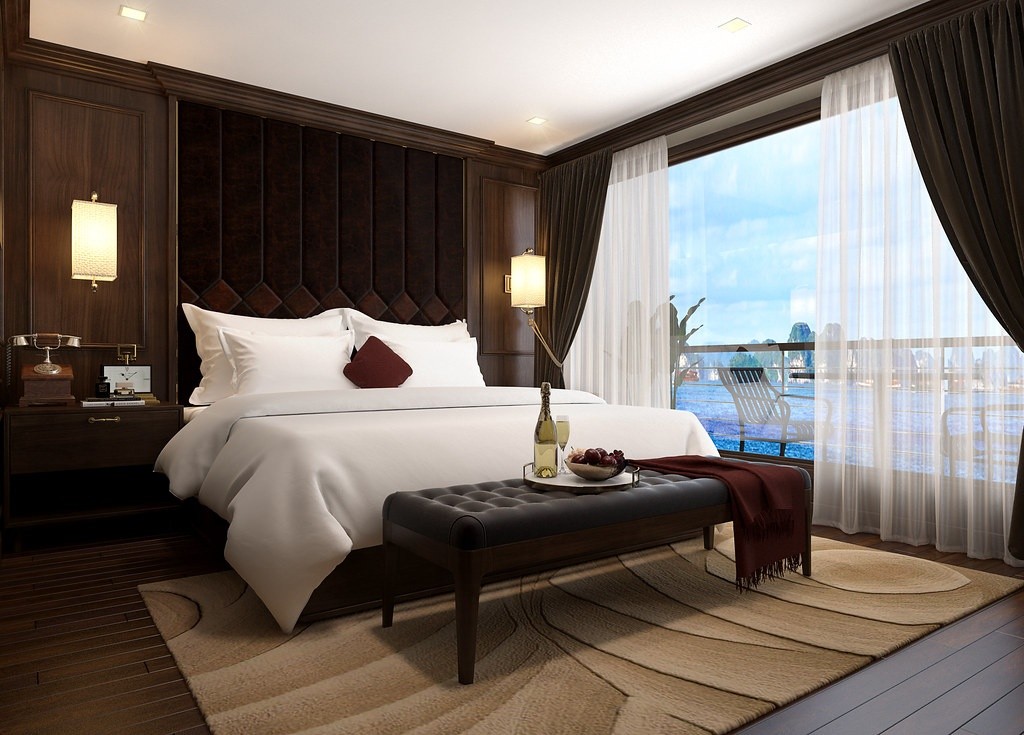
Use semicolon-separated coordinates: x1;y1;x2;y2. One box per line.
565;455;628;481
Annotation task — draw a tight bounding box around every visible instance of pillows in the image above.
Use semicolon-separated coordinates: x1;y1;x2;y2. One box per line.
366;336;486;389
218;328;360;394
354;316;470;346
181;302;345;406
344;334;412;389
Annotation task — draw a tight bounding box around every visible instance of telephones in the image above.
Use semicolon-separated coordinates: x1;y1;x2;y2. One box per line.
7;331;83;408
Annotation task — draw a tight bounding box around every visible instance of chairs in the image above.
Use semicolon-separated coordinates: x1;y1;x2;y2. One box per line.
718;367;835;461
939;404;1024;479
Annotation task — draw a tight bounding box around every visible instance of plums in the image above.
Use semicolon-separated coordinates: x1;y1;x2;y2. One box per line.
571;448;623;469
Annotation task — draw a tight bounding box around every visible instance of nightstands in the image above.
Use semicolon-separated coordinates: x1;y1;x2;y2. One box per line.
3;401;182;542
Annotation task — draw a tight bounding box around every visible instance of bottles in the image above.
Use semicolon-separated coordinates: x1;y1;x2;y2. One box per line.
534;382;558;479
96;376;111;398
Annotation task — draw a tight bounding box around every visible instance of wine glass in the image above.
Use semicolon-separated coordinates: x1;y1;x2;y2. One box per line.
555;414;571;475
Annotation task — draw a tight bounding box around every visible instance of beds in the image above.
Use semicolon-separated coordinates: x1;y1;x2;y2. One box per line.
150;304;726;629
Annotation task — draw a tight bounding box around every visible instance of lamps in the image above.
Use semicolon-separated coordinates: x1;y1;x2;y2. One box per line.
504;244;548;316
71;190;120;290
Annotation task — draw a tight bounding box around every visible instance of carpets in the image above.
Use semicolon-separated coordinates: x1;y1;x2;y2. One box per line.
137;535;1022;735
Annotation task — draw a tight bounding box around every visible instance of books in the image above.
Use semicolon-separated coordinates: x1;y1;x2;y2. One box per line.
85;396;142;402
133;393;160;405
80;400;145;408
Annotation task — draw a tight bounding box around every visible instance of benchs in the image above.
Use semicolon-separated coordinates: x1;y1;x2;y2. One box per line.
380;453;814;688
